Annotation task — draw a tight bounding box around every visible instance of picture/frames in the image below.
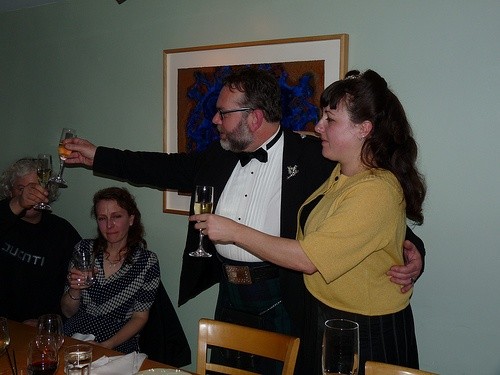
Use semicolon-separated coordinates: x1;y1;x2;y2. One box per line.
163;34;350;217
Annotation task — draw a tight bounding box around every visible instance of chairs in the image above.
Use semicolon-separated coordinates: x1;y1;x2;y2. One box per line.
196;316;301;374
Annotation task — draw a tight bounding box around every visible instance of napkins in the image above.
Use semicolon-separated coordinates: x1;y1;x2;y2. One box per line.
85;350;149;374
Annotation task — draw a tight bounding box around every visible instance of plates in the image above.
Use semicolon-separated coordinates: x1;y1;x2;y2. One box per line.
133;368;193;375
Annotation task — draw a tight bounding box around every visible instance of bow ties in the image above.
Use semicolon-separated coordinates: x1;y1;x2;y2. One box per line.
238;146;269;167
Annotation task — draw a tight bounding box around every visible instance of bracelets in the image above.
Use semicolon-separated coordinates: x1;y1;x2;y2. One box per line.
67;288;83;301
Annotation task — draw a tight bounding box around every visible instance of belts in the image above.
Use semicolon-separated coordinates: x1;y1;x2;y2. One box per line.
210;259;270;285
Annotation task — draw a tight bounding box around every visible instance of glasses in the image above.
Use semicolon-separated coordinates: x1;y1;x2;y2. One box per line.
214;107;253;119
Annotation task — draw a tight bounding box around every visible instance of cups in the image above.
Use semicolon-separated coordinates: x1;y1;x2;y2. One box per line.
63;342;92;375
76;250;96;286
27;337;60;375
0;318;12;358
321;318;360;375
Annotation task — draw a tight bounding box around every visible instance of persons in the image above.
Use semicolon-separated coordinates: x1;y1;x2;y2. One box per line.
55;185;160;358
0;158;84;333
187;66;421;375
60;68;426;375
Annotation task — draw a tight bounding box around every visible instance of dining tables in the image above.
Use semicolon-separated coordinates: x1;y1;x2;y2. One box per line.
0;314;201;374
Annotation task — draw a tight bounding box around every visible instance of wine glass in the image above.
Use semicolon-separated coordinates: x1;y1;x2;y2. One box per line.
33;155;58;211
188;185;214;257
36;314;65;363
48;128;77;186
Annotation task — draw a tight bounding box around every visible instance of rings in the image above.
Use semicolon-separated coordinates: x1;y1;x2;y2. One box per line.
410;277;415;284
